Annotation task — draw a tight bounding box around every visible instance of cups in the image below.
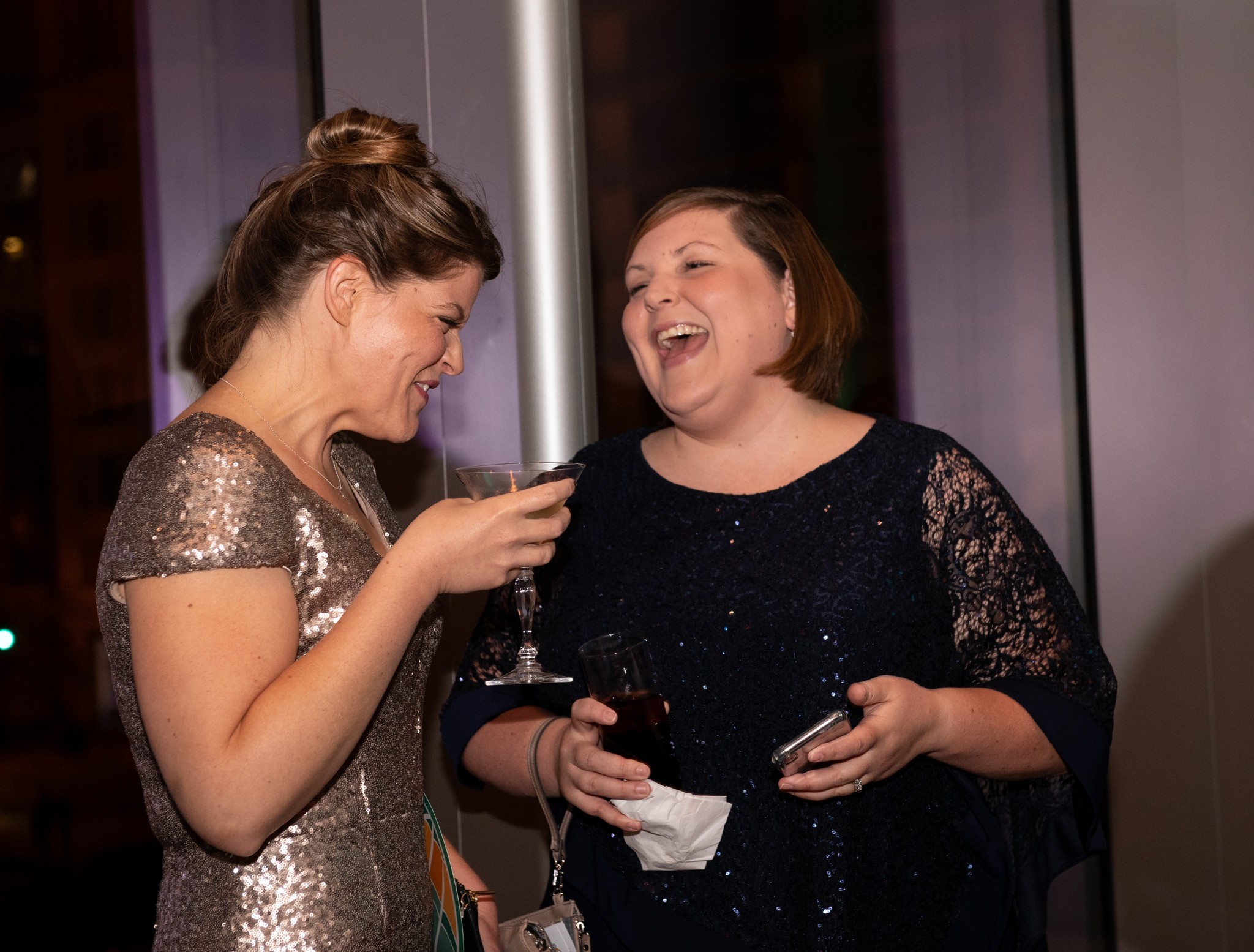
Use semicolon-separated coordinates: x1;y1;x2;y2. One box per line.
578;629;684;803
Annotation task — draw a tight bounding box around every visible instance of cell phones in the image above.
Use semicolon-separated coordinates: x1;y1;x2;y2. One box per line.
772;708;855;779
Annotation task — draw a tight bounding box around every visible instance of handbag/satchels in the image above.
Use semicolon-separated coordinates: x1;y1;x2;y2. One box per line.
497;713;592;952
455;878;496;952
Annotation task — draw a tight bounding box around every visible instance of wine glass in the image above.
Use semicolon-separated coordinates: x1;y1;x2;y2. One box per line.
452;460;587;686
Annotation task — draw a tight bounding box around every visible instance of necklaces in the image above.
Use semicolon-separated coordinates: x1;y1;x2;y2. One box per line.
220;377;350;503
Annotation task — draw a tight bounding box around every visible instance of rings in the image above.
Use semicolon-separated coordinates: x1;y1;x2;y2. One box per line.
853;778;863;792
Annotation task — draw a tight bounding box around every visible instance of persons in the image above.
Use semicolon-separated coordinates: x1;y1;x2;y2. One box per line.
95;108;575;952
438;188;1119;946
